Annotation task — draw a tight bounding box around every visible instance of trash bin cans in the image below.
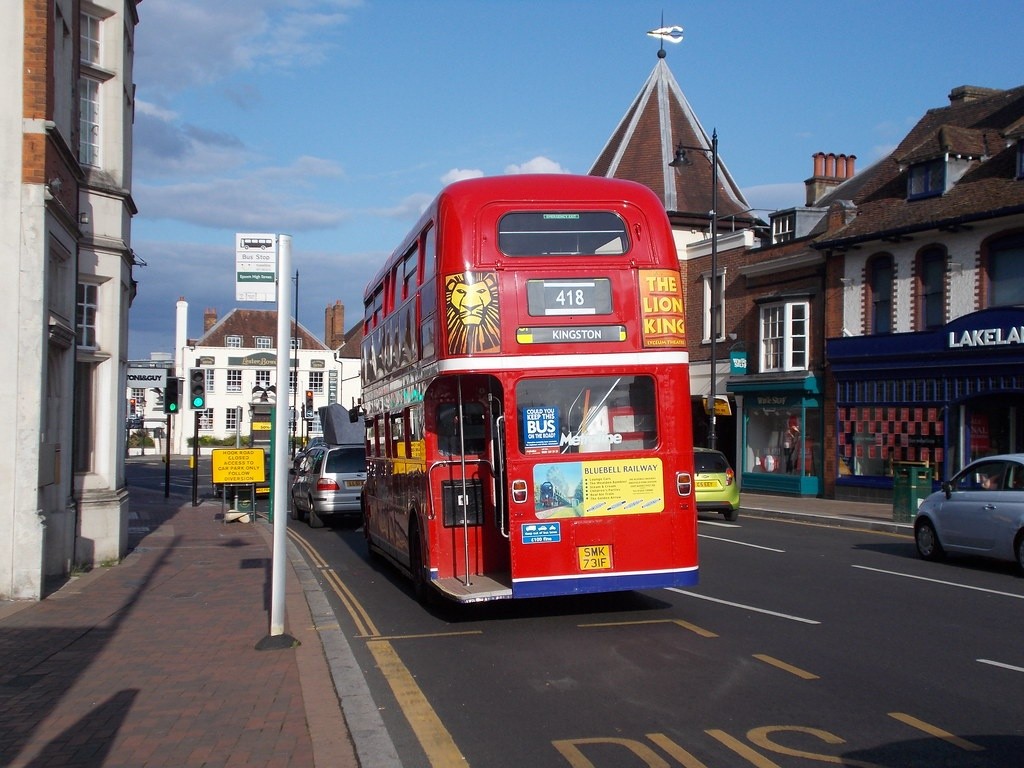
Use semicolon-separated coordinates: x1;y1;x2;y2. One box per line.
892;461;934;524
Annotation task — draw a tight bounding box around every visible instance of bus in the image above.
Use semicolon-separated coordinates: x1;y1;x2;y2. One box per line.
347;169;702;616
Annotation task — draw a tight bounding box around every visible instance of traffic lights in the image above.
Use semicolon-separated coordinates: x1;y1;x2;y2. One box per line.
186;367;208;412
162;376;180;415
304;389;315;421
129;398;137;415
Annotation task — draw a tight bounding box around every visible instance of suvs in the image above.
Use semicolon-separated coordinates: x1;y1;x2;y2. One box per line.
289;436;369;531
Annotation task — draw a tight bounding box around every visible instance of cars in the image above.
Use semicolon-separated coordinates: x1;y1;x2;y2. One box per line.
913;453;1024;571
692;447;740;523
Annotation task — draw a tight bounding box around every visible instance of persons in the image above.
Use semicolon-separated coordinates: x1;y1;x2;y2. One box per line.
783;416;800;474
982;472;999;490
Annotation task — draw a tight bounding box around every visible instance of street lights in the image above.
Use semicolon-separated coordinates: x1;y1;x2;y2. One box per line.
666;125;720;450
